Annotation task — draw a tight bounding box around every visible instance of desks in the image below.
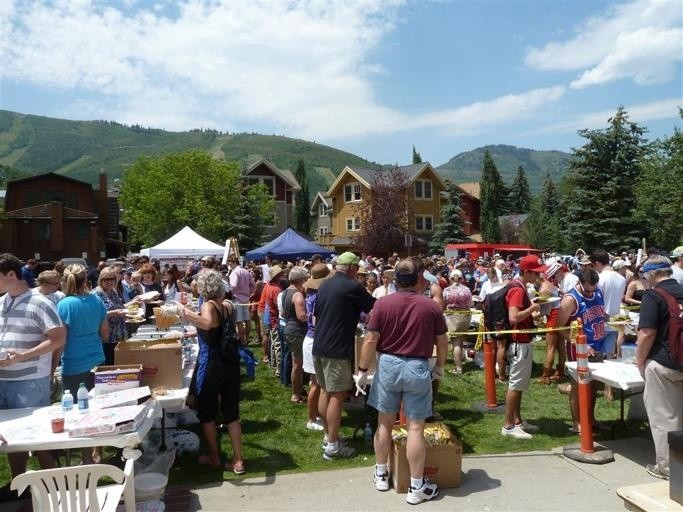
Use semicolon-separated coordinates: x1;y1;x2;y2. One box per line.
0;401;163;467
89;326;200;452
352;374;373;444
565;358;645;438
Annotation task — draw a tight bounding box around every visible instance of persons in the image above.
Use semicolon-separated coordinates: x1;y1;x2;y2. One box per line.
0;252;245;504
355;260;449;504
405;247;683;478
223;252;400;459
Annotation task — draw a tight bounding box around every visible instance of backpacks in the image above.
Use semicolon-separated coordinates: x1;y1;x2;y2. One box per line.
655;286;683;367
482;284;522;341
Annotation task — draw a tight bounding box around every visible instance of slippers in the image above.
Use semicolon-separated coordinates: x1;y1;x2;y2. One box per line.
291;390;307;403
571;421;614;437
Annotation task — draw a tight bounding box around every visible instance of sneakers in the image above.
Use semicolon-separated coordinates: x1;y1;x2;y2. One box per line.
199;455;221;468
448;368;462;374
306;416;357;460
0;482;31;501
501;419;537;439
646;463;671;481
372;466;390;491
406;477;441;505
225;461;246;475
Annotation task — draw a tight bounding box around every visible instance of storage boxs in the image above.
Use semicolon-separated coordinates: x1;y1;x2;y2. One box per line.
91;365;143;395
621;343;636;365
354;337;375;373
114;339;184;389
387;421;461;494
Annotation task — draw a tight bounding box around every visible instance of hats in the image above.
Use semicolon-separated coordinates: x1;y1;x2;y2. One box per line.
578;256;591;265
519;255;560;279
269;265;289;282
303;252;366;290
612;259;631;270
669;246;683;258
450;269;463;278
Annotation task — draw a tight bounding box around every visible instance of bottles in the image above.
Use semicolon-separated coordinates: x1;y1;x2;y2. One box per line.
61;390;74;413
365;422;372;446
77;382;89;414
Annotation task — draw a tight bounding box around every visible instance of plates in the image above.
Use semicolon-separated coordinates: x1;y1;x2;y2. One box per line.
124;319;146;324
119;310;140;314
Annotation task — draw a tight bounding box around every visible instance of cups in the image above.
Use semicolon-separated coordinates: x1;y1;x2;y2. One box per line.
50;417;65;433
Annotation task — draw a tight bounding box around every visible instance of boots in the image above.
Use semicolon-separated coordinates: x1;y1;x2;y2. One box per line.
536;366;550;384
550;364;566;381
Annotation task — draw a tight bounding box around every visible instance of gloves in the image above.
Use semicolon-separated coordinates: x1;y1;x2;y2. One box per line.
431;366;445;382
161;300;185;318
355;371;367;396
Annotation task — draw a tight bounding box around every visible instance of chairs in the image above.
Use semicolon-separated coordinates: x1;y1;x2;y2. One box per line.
10;459;136;512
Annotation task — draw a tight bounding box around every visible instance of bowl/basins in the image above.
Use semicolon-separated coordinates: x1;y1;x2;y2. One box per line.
530;296;562;307
